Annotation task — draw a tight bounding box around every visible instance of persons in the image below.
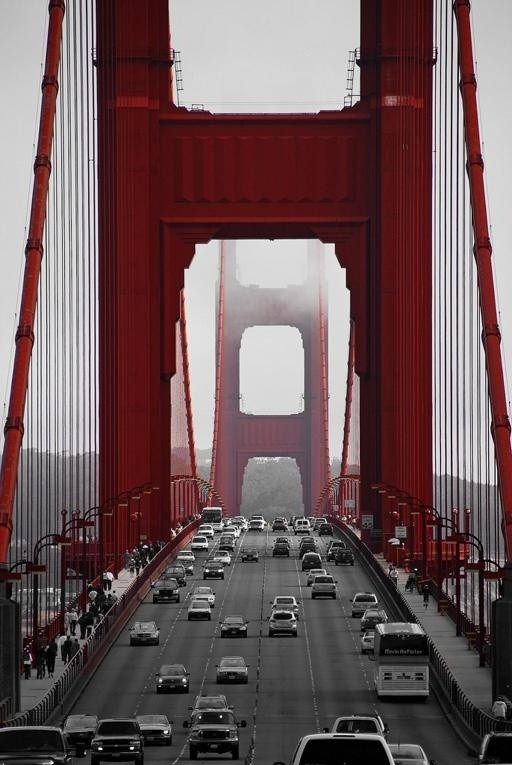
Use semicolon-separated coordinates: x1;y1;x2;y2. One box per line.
409;570;415;592
327;513;358;530
20;569;117;681
491;697;507;732
390;567;398;586
389;564;393;575
124;514;203;576
423;585;429;607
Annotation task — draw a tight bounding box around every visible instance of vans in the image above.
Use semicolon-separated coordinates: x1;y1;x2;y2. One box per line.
327;712;386;741
271;729;397;765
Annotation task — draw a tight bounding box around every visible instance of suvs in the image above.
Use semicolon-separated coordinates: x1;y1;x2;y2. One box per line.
89;716;145;765
266;610;298;637
182;709;247;762
347;591;379;618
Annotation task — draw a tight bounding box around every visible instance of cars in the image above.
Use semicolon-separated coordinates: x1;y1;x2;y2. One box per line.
128;618;162;646
187;694;235;721
219;615;249;638
213;655;251;685
148;505;355;627
155;664;191;695
63;713;101;749
358;608;388;632
1;726;76;765
358;630;376;653
136;714;175;747
386;741;430;765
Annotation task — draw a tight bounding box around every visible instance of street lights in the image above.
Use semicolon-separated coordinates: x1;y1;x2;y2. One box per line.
74;469;230;617
1;571;22;587
483;569;508;583
55;509;99;640
11;555;48;576
310;471;444;614
446;532;488;670
27;531;75;656
462;557;504;597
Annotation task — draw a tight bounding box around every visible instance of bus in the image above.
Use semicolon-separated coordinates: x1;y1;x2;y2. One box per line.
372;620;433;699
